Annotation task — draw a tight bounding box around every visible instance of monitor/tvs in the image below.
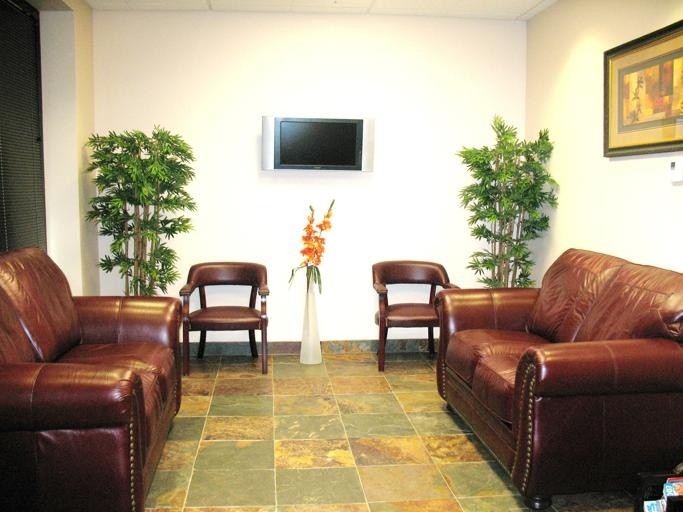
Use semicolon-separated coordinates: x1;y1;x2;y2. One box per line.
274;118;362;172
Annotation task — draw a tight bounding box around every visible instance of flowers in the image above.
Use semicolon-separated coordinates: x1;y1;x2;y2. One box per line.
286;199;335;294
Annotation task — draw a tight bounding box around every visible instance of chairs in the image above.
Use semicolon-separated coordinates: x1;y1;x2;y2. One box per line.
372;260;460;373
180;261;269;375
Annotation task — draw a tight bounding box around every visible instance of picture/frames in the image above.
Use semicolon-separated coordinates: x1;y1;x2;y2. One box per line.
603;20;683;158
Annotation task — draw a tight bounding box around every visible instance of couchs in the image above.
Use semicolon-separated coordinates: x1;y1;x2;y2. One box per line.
434;248;683;511
0;248;184;512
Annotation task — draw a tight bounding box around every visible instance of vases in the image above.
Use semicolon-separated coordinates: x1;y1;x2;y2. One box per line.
299;269;322;365
